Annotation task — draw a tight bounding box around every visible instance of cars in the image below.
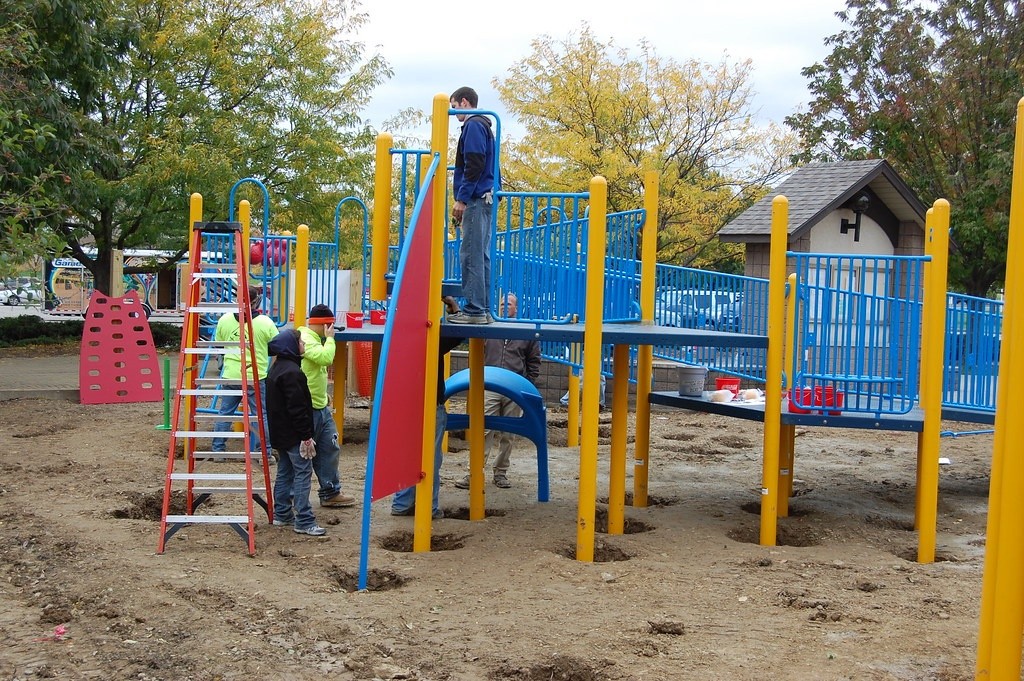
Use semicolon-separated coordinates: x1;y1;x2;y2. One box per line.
0;282;42;305
630;286;744;332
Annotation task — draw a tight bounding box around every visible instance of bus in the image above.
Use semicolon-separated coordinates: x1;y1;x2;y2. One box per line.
40;246;233;320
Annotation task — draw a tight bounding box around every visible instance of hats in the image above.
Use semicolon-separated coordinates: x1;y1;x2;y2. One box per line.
308;304;336;324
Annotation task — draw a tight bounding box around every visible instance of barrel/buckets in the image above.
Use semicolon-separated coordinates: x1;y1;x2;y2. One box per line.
788;385;844;416
715;377;741;400
677;363;707;396
346;312;363;328
370;310;387;325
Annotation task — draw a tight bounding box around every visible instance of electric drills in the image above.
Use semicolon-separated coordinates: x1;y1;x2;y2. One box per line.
552;313;579;324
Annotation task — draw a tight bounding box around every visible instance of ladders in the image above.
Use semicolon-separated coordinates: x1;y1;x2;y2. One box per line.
155;217;276;557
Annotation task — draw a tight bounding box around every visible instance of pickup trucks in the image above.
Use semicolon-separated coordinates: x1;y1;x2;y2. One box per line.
0;276;41;290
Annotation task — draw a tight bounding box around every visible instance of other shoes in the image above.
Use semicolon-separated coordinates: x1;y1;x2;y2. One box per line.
259;456;276;465
213;458;225;462
391;507;415;516
432;510;444;521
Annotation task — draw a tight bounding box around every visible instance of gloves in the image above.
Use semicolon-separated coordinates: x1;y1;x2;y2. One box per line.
300;438;316;459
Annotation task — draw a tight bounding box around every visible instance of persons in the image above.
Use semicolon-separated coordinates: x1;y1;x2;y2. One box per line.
211;284;281;466
559;342;607;413
265;328;327;535
289;303;357;507
454;291;542;490
391;295;468;518
447;86;503;325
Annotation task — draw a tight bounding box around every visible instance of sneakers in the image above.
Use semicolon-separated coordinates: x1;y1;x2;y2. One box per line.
492;476;511;488
454;475;470;488
294;524;327;535
447;314;487;324
273;517;296;526
486;312;494;323
322;491;355;506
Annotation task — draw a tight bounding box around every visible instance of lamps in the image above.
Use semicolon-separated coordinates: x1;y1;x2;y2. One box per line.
840;190;871;242
